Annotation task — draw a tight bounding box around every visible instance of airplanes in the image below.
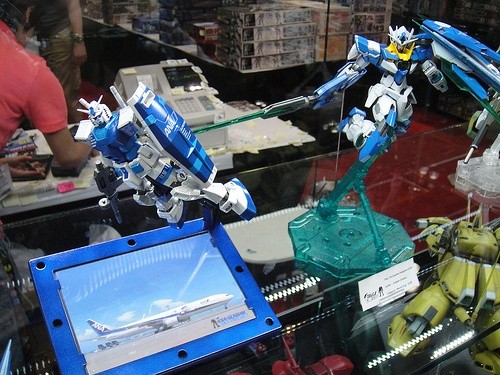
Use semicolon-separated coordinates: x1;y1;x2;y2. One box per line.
81;293;235;344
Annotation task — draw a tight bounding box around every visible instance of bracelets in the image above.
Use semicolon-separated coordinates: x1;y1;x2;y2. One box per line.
72;33;84;43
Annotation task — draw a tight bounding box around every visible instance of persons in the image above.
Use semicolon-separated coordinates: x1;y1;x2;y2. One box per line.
0;0;97;180
20;0;88;122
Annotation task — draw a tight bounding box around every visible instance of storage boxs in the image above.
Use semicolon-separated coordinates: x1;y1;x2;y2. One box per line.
215;0;393;71
